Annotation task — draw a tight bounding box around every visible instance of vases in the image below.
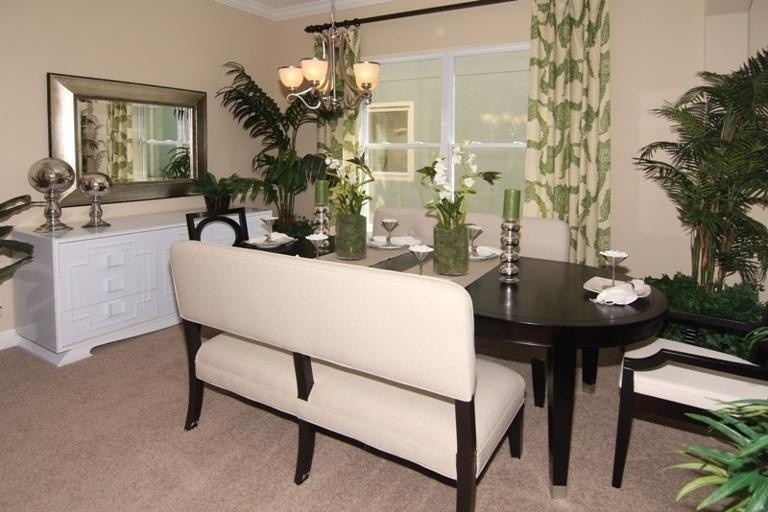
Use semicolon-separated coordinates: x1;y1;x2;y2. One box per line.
433;227;468;276
334;215;366;261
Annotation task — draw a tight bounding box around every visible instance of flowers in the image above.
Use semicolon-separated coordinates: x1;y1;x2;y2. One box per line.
411;141;501;228
319;143;382;216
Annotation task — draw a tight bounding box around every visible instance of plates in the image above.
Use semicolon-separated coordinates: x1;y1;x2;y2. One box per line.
584;276;651;299
245;233;295;248
467;247;502;259
368;235;412;249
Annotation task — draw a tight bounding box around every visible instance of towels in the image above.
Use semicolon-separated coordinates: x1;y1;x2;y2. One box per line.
477;246;503;256
590;279;645;305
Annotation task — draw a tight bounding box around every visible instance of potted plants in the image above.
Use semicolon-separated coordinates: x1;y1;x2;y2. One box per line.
636;53;764;365
226;60;325;233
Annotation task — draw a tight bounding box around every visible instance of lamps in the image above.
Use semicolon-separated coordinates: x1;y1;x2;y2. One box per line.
278;4;382;117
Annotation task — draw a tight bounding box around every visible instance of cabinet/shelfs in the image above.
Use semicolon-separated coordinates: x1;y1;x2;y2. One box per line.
10;206;276;369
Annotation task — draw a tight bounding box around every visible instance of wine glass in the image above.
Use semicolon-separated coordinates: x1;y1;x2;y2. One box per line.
260;217;279;244
305;234;329;261
467;227;484;255
600;251;629;291
380;220;399;247
409;246;434;276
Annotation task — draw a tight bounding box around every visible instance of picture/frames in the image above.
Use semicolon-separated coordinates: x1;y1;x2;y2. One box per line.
46;73;213;209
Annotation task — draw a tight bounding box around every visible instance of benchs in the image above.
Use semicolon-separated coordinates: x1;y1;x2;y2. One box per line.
165;240;530;512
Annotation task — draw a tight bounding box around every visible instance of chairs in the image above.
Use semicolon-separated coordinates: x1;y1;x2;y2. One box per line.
609;319;764;488
